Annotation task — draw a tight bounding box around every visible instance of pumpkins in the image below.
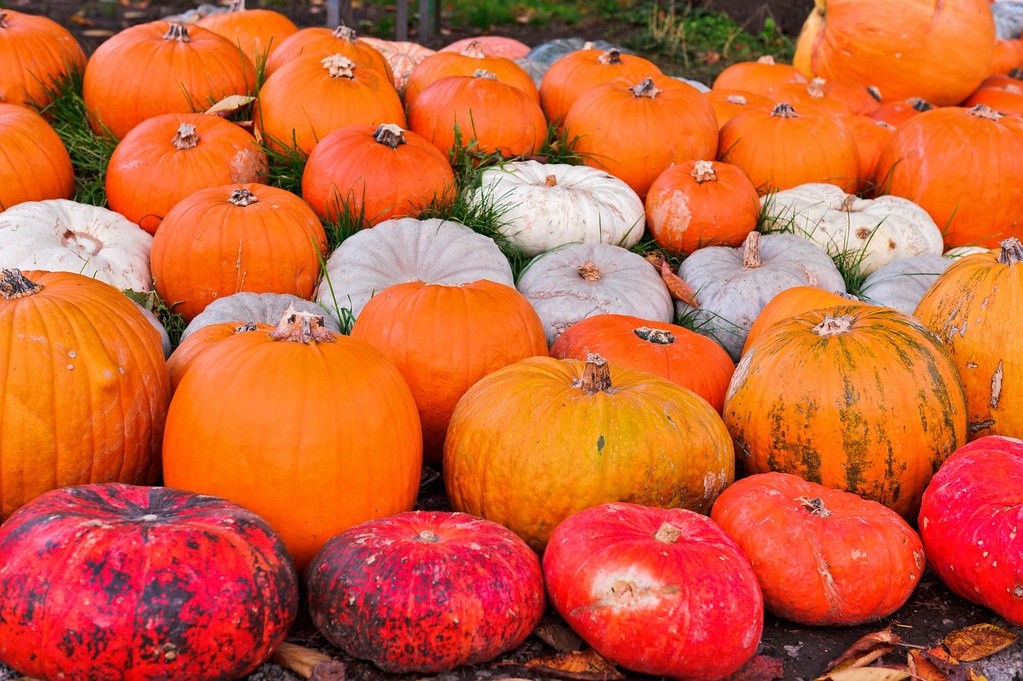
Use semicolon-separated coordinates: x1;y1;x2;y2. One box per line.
0;0;1023;681
